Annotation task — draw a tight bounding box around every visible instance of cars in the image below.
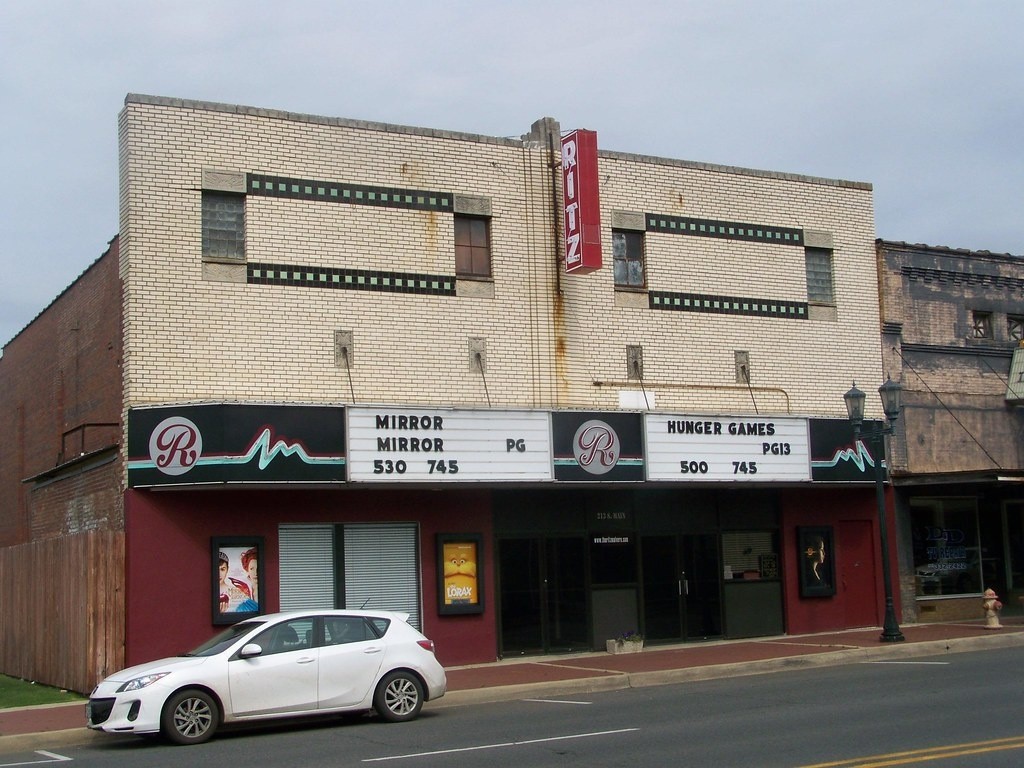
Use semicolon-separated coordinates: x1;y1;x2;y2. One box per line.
85;609;449;747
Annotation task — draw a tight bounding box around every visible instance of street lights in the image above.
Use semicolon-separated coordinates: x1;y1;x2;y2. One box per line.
843;373;906;643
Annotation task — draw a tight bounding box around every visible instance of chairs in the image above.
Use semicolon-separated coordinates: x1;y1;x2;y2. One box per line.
280;624;313;651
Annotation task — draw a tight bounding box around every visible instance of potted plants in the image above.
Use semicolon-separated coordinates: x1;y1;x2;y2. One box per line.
742;547;760;580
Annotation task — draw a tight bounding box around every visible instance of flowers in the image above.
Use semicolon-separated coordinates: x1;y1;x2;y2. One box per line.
608;630;645;645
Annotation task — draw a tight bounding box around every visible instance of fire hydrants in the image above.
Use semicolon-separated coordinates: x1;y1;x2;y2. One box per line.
982;586;1003;630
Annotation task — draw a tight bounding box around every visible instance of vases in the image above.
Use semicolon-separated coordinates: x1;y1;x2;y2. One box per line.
607;639;644;655
